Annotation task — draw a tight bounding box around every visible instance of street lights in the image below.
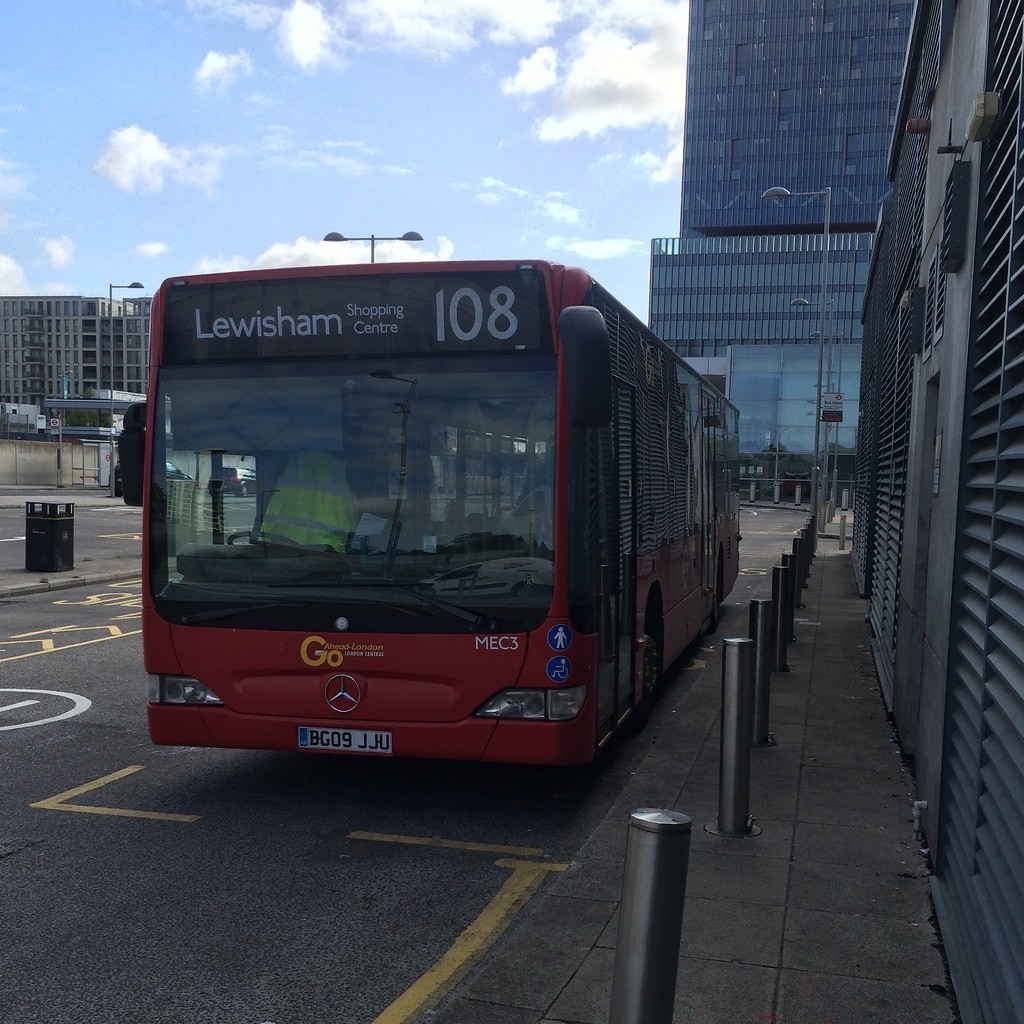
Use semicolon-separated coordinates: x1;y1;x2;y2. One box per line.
806;382;834;511
109;282;145;428
791;297;839;521
809;331;844;525
322;230;424;263
759;184;832;556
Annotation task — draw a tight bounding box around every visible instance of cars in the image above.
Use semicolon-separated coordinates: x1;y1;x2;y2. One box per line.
207;467;257;498
107;460;196;497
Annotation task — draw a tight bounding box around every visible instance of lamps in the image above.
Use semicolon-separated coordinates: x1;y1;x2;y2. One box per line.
965;91;999;144
899;290;913;309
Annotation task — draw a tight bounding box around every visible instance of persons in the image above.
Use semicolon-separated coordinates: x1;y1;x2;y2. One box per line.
257;426;468;554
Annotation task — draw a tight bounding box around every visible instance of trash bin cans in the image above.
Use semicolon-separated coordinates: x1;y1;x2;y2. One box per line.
25;499;75;573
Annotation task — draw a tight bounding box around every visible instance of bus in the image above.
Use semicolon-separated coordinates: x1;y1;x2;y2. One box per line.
112;260;741;784
